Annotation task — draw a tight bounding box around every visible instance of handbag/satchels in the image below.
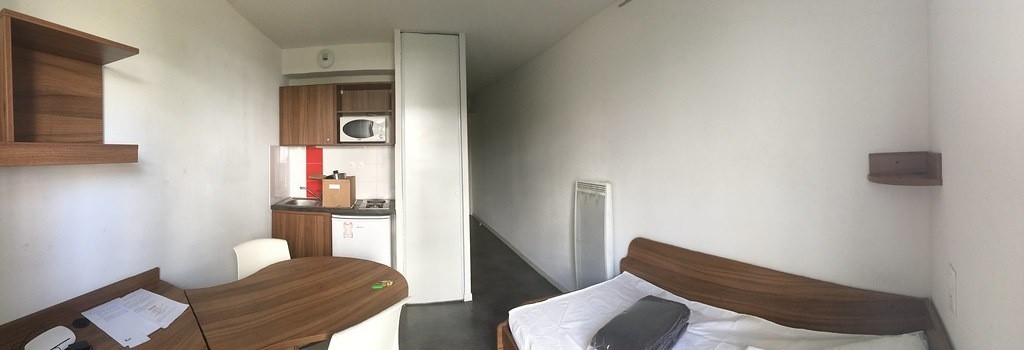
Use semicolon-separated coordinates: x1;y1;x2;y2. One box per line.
590;294;690;350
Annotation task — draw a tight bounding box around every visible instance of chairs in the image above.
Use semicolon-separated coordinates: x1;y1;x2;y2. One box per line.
234;237;292;281
326;296;413;350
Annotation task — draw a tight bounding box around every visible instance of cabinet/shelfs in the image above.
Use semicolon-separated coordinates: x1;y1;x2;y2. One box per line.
280;82;396;147
272;211;332;260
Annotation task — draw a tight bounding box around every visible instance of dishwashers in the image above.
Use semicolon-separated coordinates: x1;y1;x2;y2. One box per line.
331;214;392;267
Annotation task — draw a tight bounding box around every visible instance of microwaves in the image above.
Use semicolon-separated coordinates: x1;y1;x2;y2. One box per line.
340;114;391;144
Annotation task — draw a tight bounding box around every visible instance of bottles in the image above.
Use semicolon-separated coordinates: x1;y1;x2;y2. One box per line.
333;170;338;180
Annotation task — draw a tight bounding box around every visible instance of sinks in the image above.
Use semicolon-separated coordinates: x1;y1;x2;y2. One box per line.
277;199;320;207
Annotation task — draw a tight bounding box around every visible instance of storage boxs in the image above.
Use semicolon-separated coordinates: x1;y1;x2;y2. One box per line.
309;174;356;207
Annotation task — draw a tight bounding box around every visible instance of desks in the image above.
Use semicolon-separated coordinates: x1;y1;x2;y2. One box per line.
0;255;410;350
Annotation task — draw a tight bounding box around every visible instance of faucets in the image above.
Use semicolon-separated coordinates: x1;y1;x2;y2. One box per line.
300;186;322;201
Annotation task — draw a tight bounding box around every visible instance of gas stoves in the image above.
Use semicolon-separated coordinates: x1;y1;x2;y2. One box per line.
358;198;390;209
